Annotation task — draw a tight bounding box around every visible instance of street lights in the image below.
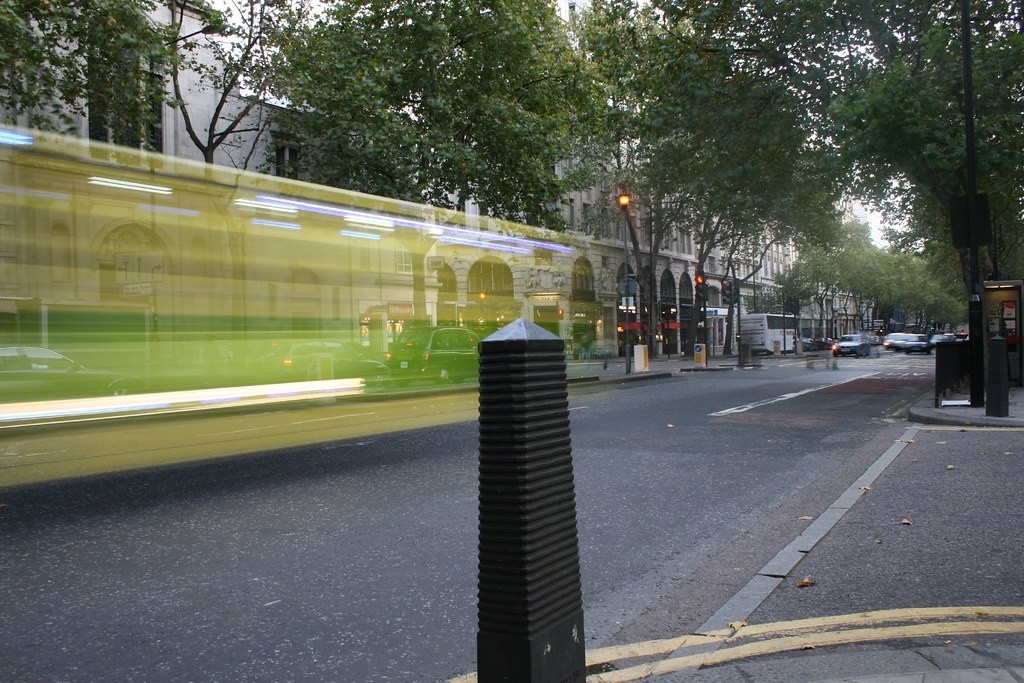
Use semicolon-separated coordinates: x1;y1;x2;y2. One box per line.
619;191;635;373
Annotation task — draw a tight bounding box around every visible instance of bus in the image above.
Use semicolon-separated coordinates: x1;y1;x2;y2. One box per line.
739;314;799;354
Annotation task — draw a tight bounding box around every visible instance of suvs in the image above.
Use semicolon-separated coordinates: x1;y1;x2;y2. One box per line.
385;326;479;386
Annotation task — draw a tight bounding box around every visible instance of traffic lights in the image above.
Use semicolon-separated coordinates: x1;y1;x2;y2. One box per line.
790;299;800;315
695;272;705;303
721;279;734;306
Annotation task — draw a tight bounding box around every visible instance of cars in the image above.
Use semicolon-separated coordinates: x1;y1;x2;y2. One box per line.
800;337;835;350
0;345;143;398
274;341;386;379
929;332;969;347
832;333;871;357
906;333;932;354
870;332;908;349
